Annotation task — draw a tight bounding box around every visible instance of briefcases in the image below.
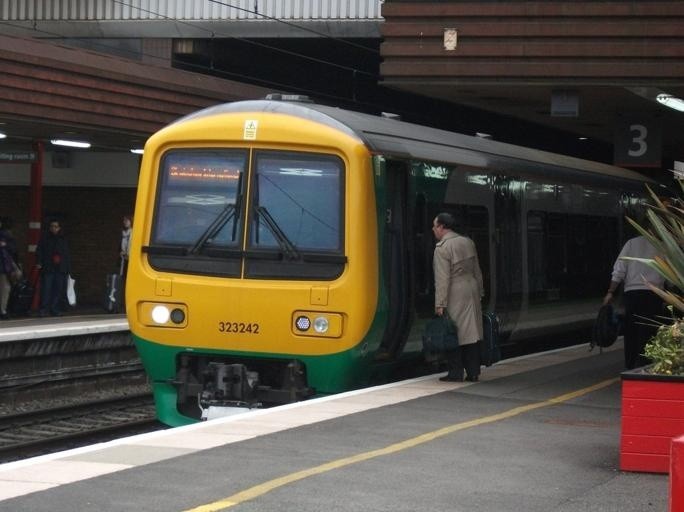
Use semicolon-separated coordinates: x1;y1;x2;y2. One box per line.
479;312;501;367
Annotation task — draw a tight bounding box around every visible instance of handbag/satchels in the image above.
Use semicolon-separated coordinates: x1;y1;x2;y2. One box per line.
421;310;459;354
14;269;23;280
67;275;77;306
595;303;617;347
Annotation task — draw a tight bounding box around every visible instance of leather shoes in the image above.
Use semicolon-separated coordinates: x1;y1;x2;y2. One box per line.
466;375;478;382
439;375;462;382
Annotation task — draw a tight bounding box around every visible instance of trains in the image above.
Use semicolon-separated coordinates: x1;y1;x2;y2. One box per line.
123;93;683;428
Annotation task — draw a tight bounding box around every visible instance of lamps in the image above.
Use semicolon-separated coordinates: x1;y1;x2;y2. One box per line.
656;93;684;112
443;28;457;52
50;139;91;149
130;148;144;154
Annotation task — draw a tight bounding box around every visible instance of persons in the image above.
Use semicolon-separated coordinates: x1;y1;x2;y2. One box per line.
118;213;132;285
428;211;485;383
0;226;69;274
604;219;674;370
33;218;74;316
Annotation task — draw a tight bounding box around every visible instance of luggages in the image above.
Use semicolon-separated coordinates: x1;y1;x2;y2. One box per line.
5;269;41;320
99;256;125;313
